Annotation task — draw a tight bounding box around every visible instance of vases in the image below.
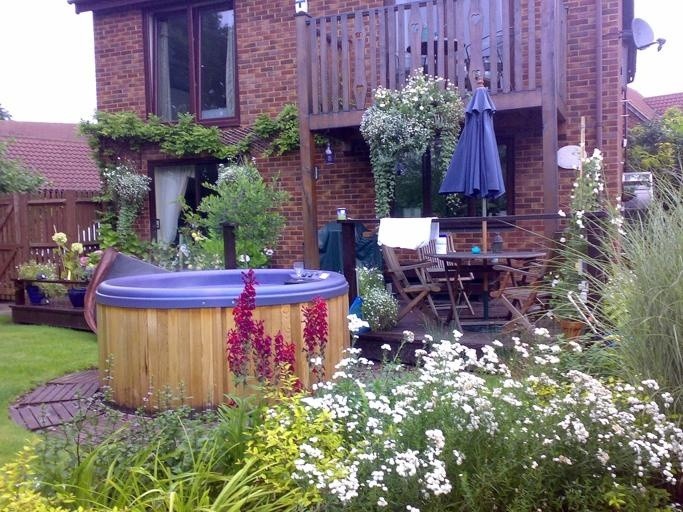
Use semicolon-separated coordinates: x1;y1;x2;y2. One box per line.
67;288;87;308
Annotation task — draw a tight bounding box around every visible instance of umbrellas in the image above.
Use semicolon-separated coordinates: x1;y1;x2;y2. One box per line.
437;78;508;322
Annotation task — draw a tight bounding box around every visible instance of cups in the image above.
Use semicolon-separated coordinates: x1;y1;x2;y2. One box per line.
337;207;347;220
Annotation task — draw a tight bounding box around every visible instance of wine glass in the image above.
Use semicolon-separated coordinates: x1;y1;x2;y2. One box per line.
293;261;304;282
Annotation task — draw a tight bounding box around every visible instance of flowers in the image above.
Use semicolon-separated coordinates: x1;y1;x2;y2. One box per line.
559;147;627;235
353;68;469;219
50;230;102;286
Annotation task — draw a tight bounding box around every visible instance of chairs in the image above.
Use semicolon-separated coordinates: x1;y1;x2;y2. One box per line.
377;229;570;335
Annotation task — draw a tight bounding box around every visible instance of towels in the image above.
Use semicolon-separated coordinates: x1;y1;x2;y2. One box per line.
376;216;440;251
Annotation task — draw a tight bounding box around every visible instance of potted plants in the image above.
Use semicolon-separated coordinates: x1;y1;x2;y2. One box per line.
16;261;56;305
562;303;585;338
